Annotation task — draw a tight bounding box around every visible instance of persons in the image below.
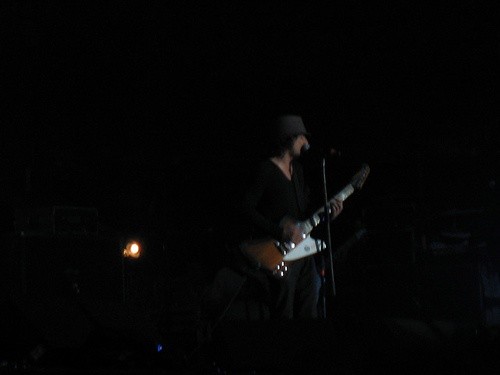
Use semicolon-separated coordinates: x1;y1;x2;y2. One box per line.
240;115;344;319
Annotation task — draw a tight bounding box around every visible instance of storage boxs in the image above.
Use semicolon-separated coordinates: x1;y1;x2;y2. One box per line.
14;206;100;236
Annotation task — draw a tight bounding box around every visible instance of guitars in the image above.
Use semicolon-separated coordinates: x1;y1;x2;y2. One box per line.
241;163;371;281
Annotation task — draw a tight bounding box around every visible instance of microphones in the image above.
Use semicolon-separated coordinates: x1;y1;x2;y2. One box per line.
303;144;311;152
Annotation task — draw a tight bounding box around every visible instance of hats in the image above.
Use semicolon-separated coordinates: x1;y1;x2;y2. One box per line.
268;115;308;137
